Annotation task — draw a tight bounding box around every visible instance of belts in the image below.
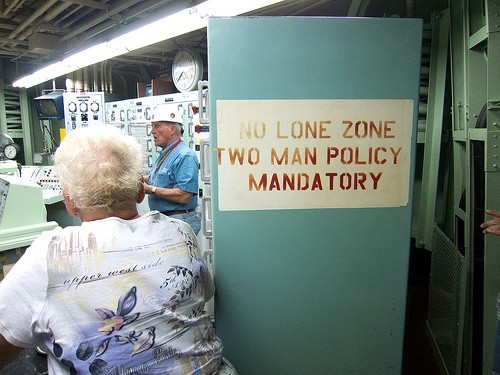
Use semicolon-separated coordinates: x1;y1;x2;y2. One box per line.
160;209;194;216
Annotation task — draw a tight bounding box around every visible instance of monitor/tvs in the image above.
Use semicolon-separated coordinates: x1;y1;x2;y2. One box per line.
34;92;64;120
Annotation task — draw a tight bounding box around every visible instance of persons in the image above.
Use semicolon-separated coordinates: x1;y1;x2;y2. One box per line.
0;122;238;375
141;104;201;241
480;210;500;236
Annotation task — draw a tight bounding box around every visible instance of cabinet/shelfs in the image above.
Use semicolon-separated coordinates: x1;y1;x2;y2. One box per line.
449;0;500;375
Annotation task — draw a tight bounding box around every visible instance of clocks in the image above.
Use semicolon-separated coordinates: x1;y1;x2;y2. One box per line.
172;48;203;93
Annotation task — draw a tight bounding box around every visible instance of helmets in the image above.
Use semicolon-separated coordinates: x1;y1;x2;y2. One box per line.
150;104;184;125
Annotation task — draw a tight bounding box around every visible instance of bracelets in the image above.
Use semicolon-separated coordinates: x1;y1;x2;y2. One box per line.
152;186;156;194
